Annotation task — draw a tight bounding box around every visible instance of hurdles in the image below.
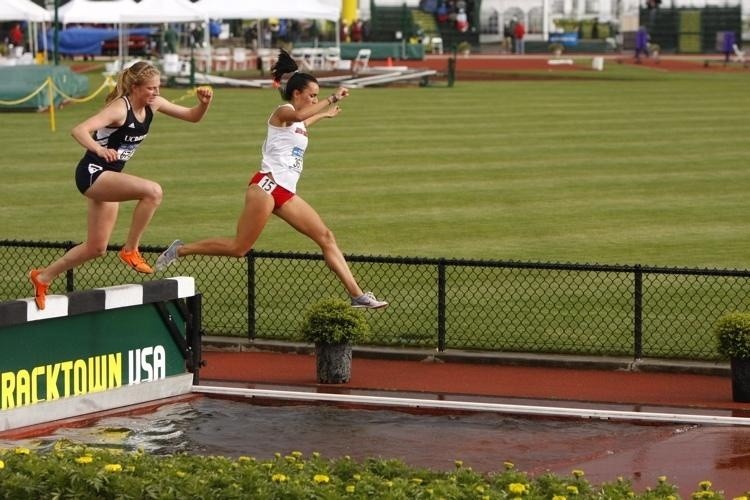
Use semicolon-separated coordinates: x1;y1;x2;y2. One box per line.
0;276;201;432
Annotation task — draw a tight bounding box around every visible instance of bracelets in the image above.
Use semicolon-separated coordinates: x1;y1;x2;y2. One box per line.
326;93;338;105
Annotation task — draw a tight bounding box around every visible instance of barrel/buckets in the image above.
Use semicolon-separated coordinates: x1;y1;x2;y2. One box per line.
592;56;604;71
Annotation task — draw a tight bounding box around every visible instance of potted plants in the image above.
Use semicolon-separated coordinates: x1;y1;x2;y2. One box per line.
715;311;750;402
300;298;372;385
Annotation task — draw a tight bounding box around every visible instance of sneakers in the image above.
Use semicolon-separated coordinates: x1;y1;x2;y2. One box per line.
29;270;50;310
156;239;184;270
118;248;154;274
351;294;389;309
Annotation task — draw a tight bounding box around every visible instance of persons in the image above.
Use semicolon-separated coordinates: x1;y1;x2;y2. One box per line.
437;1;469;32
28;62;212;310
9;23;24;45
635;25;650;64
151;23;363;48
156;48;388;309
502;19;525;55
723;31;737;61
448;58;455;87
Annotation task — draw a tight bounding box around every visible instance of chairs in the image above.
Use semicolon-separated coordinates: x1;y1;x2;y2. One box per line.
197;46;375;73
